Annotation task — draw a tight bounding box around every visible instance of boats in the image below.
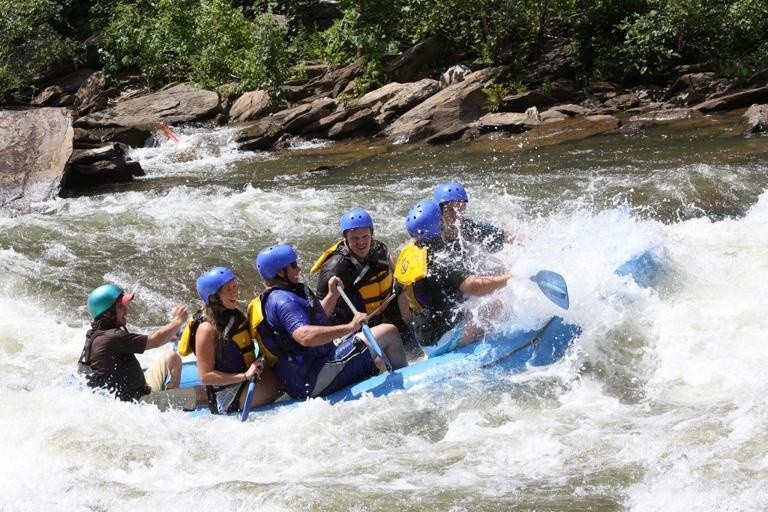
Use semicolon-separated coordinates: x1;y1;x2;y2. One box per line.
141;245;663;423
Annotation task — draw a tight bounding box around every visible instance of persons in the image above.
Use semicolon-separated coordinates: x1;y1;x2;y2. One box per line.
433;182;528;259
77;284;209;411
179;267;284;411
246;244;408;400
311;208;410;335
391;199;512;357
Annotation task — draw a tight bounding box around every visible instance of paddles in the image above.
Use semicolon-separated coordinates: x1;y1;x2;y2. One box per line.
242;356;264;423
336;284;392;369
486;255;569;310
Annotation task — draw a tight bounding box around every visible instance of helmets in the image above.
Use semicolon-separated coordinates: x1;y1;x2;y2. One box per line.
88;285;134;321
340;209;373;235
196;267;236;305
256;244;298;281
405;183;468;244
